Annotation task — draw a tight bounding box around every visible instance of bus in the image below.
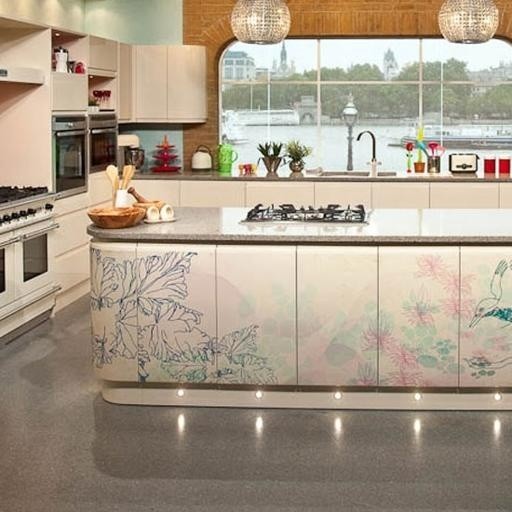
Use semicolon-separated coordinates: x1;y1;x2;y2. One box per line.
236;109;300;126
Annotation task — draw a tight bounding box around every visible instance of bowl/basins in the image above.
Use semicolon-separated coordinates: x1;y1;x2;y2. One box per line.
88;207;145;229
134;201;163;211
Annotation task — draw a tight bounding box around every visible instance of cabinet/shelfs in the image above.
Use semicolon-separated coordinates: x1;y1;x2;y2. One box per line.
50;199;114;316
429;181;500;209
245;180;314;209
0;16;208;123
314;180;372;209
116;180;180;208
500;181;512;209
180;180;246;208
372;180;430;209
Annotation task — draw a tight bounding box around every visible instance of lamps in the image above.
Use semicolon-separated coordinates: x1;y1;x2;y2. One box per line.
231;0;291;45
438;0;499;44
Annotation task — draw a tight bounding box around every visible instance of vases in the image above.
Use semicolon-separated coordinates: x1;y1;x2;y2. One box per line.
289;159;305;173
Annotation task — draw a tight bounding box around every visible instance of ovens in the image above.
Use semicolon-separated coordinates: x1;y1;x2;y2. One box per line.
0;220;62;347
89;114;118;174
51;112;88;202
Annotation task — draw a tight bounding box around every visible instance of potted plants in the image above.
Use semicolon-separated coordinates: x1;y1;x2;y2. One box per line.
258;141;284;174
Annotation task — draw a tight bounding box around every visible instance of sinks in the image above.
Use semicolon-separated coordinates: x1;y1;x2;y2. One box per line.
319;171;396;177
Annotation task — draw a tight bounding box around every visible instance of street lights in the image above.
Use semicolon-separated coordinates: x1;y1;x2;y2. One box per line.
342;94;358;171
440;60;448;146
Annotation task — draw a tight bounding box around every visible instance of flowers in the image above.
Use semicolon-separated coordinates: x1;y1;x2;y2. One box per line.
283;140;312;160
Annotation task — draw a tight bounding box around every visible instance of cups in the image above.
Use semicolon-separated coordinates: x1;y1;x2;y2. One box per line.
239;163;257;175
484;155;510;174
115;189;127;206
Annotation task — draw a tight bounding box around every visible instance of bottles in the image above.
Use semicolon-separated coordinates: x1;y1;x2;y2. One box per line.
54;46;68;72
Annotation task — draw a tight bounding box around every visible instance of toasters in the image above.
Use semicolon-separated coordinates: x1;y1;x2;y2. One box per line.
450;153;478;173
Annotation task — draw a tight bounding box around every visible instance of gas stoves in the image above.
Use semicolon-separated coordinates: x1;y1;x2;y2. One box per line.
0;186;55;233
245;203;366;224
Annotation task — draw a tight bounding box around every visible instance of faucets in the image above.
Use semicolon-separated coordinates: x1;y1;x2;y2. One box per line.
356;131;376;159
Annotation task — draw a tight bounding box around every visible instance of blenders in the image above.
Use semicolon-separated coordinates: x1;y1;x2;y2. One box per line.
119;134;145;171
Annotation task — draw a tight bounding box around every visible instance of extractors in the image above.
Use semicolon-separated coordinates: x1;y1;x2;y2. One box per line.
0;64;46;85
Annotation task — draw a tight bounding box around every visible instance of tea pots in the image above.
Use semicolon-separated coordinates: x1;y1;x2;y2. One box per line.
191;143;238;173
